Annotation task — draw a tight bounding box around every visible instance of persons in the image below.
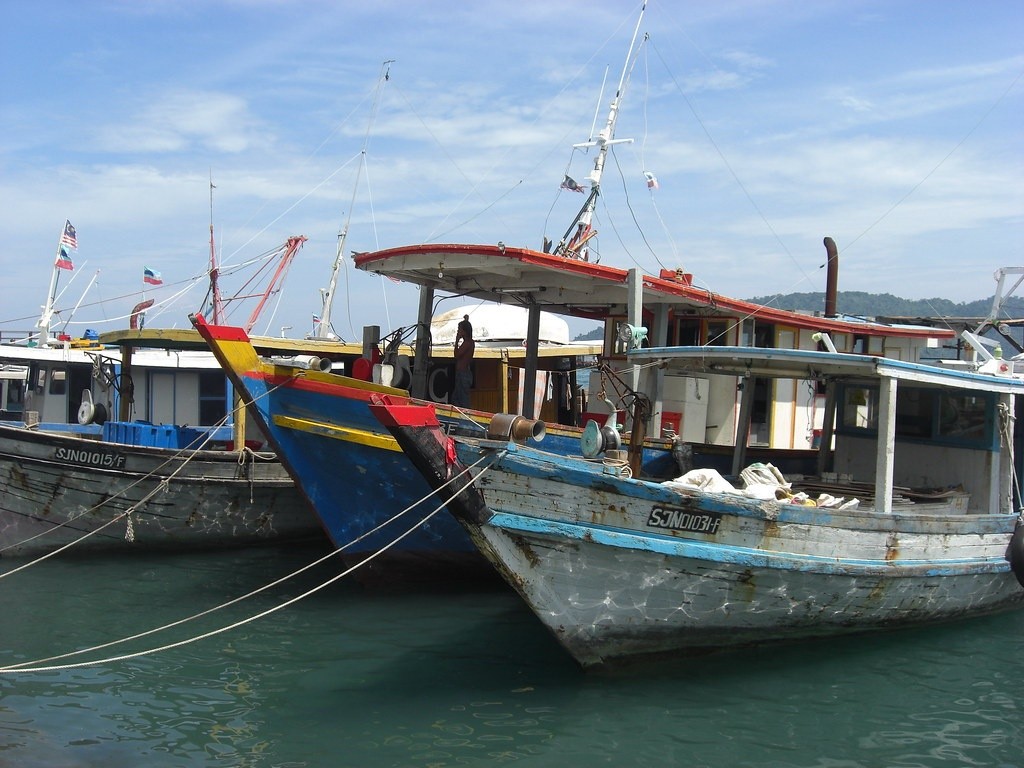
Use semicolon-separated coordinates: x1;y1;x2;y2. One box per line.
453;321;475;407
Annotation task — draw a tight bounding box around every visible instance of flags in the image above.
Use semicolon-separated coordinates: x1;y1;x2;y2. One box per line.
60;219;78;249
142;267;163;285
55;244;75;270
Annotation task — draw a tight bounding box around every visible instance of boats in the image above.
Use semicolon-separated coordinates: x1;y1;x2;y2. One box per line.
0;0;1023;672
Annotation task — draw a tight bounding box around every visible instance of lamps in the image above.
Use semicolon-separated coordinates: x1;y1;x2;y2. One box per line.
619;324;648;349
812;331;822;343
499;241;507;254
994;344;1003;360
